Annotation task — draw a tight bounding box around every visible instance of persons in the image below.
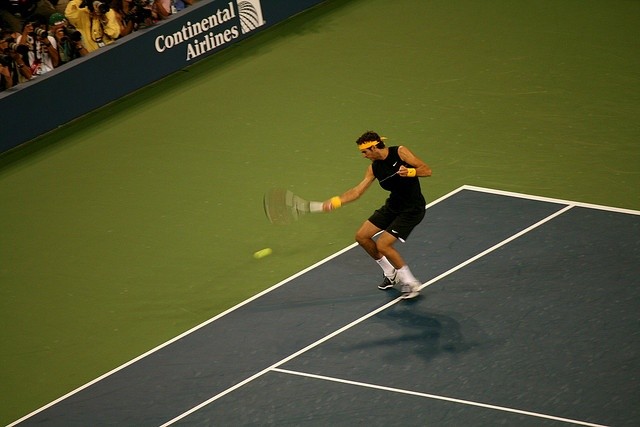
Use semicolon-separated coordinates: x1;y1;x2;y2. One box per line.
0;1;191;93
323;130;432;299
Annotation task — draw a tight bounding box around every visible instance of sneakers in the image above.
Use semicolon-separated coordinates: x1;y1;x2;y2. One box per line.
400;281;421;299
378;269;399;290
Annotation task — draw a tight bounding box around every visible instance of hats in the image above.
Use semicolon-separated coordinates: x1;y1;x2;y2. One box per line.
49;13;65;25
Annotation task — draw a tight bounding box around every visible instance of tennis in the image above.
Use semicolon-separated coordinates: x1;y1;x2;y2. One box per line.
252;248;271;260
